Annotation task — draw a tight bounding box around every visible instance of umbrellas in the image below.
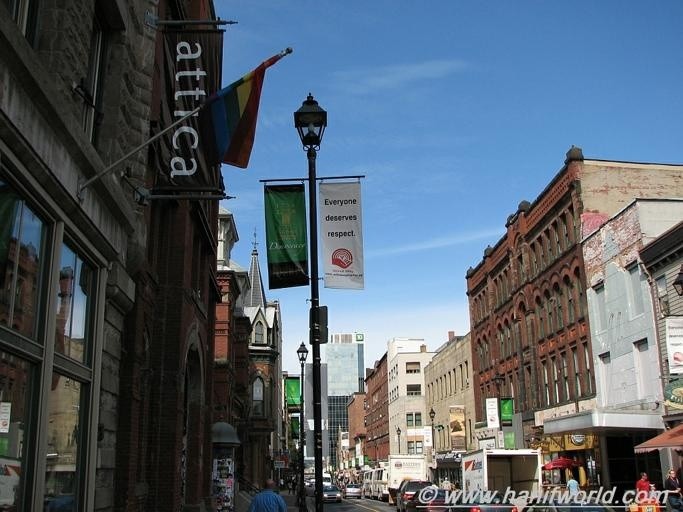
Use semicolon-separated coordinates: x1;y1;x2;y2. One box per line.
541;456;584;470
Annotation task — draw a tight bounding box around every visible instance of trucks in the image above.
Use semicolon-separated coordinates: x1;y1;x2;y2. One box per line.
387;454;434;506
460;447;543;504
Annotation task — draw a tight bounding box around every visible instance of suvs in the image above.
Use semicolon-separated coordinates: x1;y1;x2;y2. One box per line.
342;483;361;499
396;478;433;512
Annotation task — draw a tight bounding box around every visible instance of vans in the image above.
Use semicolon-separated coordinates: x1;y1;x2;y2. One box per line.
322;471;332;487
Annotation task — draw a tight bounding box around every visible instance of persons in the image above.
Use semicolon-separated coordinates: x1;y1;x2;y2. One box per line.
247;477;287;512
441;477;452;490
664;469;683;511
565;474;581;499
635;472;655;498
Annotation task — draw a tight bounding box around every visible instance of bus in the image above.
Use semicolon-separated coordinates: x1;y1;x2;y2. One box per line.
362;467;389;504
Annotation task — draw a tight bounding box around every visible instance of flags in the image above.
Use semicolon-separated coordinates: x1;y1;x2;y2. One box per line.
290;416;299;439
448;404;466;453
284;377;300;413
485;397;500;428
198;51;283;169
263;184;308;290
318;182;364;290
499;397;513;426
146;28;224;195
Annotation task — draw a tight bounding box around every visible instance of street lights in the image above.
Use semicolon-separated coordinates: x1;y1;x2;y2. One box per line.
429;408;436;486
292;92;328;512
396;426;401;455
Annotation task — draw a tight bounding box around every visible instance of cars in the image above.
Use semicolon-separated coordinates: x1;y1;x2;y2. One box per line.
441;489;616;512
405;488;453;512
322;484;342;504
303;478;316;497
